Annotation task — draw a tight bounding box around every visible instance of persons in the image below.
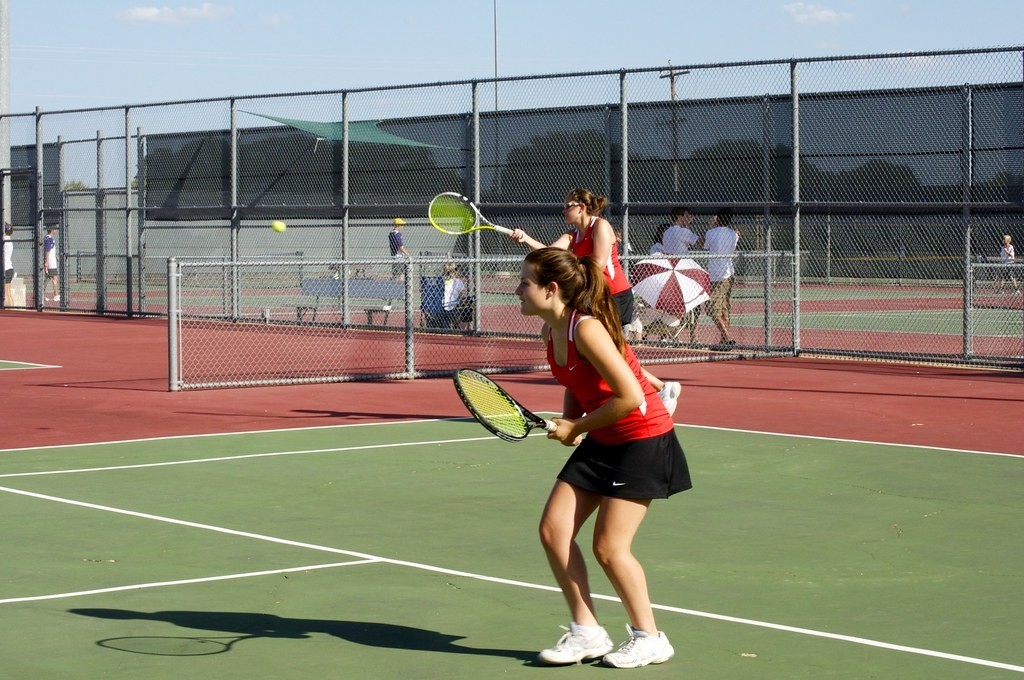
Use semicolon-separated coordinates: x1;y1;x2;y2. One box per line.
995;235;1022;295
3;222;15;309
702;213;740;351
42;223;61;302
649;223;671;256
661;207;706;349
514;247;694;670
508;187;633;327
388;217;410;301
442;262;474;330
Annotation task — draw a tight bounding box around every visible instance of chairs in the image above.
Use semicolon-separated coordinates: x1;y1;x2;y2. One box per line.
419;276;469;330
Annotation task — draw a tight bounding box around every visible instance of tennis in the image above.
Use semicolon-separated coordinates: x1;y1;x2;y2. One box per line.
272;221;286;232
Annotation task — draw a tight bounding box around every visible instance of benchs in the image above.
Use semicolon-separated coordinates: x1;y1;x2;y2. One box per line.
284;276;405;325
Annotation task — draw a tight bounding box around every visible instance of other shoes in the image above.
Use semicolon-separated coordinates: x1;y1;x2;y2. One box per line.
45;294;61;302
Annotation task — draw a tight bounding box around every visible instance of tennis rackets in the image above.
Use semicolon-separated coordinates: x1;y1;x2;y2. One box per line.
429;191;527;243
453;368;582;445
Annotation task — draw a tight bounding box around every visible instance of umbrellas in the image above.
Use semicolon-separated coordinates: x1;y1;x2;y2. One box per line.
632;251;712;319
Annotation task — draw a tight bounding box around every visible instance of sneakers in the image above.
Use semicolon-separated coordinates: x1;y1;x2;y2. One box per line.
602;627;675;668
537;622;615;664
657;381;682;417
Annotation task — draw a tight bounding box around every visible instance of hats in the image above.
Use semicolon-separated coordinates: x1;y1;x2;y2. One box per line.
393;218;406;224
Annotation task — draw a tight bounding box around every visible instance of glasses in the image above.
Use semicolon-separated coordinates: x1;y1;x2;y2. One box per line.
562;201;580;209
